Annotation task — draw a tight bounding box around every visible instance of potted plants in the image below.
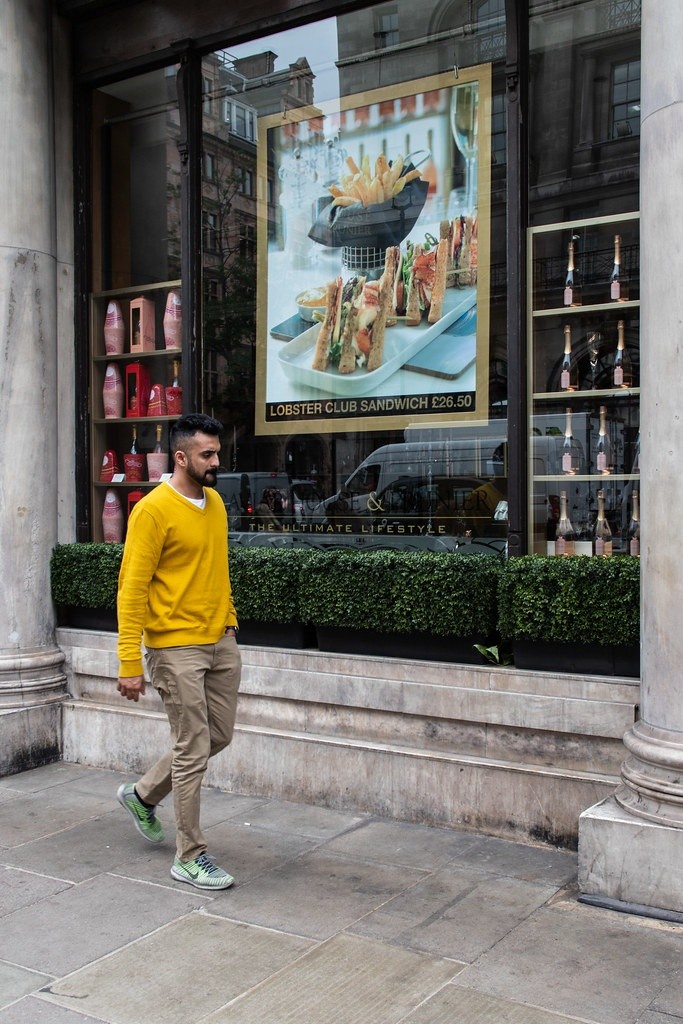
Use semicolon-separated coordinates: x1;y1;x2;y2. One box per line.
51;542;640;679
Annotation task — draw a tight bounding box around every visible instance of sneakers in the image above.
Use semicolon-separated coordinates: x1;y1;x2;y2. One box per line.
117;783;165;843
170;851;235;890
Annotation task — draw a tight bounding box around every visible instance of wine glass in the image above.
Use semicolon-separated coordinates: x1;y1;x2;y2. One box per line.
587;332;602;390
450;82;478;213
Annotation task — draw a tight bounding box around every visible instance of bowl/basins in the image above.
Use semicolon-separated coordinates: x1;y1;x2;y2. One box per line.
295;288;326;322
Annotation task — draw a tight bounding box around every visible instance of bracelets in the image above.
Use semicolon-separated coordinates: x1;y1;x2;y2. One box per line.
226;626;239;631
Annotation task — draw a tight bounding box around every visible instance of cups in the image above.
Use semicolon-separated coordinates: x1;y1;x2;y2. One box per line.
165;387;182;416
124;454;145;482
448;188;477;223
147;453;169;483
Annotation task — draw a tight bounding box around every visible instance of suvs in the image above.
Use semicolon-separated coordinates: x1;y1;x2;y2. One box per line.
291;478;323;522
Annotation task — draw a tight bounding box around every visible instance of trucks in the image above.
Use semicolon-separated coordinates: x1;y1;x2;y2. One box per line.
402;410;626;518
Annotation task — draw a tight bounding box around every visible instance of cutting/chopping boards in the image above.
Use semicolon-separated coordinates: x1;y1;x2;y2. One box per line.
270;283;478;381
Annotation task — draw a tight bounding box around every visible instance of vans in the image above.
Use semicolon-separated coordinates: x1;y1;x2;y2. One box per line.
312;436;585;528
213;472;294;532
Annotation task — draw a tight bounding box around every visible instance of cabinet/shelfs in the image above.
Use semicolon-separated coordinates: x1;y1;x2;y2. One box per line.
88;279;183;547
528;211;640;561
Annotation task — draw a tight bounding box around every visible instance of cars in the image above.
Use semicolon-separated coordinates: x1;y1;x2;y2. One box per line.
359;475;508;538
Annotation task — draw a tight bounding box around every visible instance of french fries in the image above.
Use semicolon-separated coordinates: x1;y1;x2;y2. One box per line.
327;152;422;206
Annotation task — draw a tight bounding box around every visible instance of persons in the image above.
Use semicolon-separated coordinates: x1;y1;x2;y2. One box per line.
115;413;242;891
359;473;379;493
464;443;509;531
433;482;459;537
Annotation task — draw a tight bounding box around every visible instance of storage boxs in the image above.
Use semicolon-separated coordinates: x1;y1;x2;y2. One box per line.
125;365;150;417
130;297;157;354
127;490;146;515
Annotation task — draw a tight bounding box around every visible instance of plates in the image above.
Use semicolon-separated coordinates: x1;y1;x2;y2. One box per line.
279;286;478;397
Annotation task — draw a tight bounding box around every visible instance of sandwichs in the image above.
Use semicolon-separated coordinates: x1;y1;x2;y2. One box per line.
310;215;478;374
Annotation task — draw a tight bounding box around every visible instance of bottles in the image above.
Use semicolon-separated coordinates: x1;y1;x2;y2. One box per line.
134;295;145;344
631;428;641;474
560;326;579;392
153;424;165;454
128;425;141;455
313;139;348;258
555;491;575;557
279;147;320;266
172;360;181;388
129;359;137;410
559;235;629;306
593;491;612;558
626;490;640;557
561;405;617;476
613;320;632;389
